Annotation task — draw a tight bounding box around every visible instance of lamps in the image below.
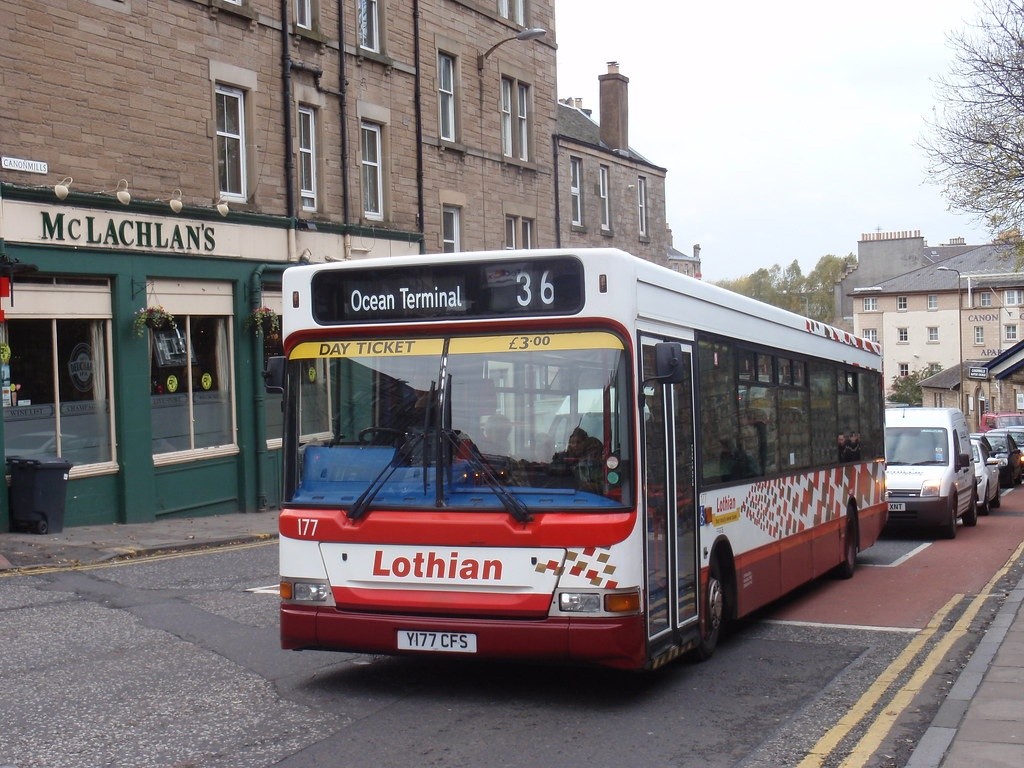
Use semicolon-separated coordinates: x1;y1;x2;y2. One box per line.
94;178;131;205
154;189;183;214
205;194;230;218
34;177;74;200
476;28;547;70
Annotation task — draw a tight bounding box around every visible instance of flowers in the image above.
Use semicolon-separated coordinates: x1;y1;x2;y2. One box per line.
244;306;280;338
131;304;178;337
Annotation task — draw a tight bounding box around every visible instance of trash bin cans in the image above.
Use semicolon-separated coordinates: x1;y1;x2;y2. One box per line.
6;455;73;535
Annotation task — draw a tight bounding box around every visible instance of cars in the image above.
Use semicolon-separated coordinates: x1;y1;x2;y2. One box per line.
969;409;1024;515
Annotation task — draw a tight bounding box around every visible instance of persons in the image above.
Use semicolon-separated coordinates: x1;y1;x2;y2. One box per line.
838;432;860;462
567;428;604;479
479;413;511;456
373;374;436;445
535;433;556;463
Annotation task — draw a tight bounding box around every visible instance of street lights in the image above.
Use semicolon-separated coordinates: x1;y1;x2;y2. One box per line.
936;265;964;413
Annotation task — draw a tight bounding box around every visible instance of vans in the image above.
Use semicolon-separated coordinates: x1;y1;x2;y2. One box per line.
882;406;979;540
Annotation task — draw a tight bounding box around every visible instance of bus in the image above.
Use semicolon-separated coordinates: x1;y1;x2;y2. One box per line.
261;247;888;671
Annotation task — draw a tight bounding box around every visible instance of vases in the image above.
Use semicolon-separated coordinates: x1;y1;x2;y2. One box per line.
145;317;169;331
256;316;272;331
